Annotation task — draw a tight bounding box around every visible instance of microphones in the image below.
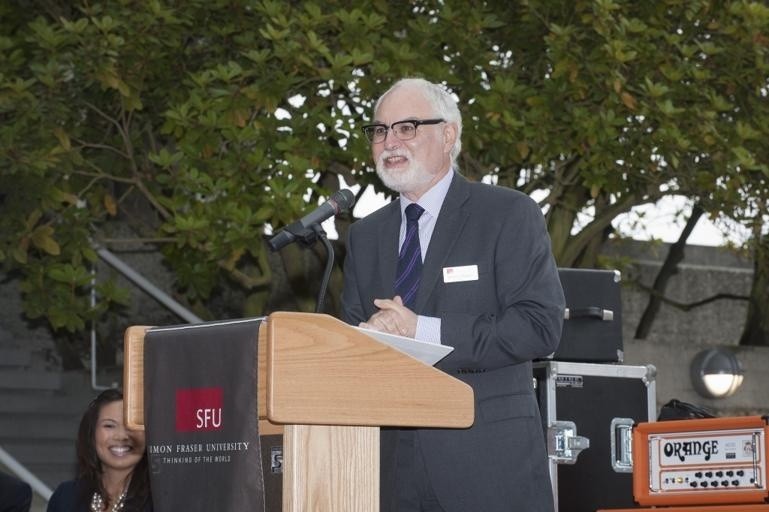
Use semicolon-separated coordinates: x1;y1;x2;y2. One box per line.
267;188;356;252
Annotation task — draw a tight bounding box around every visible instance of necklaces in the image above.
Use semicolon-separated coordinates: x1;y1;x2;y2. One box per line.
92;490;128;512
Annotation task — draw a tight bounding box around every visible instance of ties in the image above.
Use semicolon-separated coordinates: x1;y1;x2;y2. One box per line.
393;204;425;309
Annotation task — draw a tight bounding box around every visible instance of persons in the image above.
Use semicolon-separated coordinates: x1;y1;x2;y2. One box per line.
0;470;32;512
46;388;153;512
338;79;567;512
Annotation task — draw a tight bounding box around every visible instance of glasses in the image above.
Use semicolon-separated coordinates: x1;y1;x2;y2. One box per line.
361;120;445;145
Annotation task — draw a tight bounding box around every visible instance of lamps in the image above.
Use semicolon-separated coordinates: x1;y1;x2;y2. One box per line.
701;346;746;398
